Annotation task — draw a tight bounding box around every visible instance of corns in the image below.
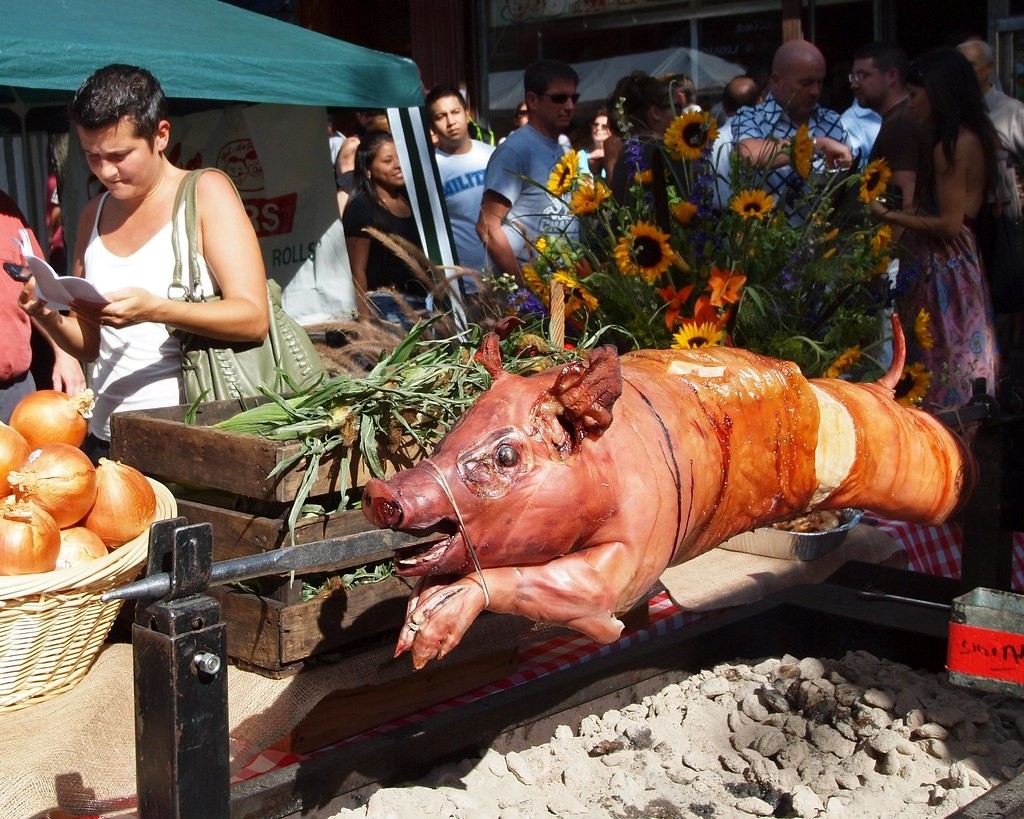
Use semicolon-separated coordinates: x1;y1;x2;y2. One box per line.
201;344;470;439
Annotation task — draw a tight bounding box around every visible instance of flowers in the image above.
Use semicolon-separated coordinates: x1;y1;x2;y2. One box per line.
479;79;938;407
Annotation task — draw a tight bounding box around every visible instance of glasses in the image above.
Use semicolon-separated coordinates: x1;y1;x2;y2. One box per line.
849;67;883;82
534;92;580;103
660;102;683;115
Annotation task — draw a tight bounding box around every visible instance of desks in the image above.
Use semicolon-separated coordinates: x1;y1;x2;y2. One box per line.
36;474;1024;819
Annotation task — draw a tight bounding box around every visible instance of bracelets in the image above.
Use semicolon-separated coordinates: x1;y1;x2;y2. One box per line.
813;137;816;144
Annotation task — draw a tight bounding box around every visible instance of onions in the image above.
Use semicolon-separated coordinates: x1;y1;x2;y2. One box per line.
0;389;156;577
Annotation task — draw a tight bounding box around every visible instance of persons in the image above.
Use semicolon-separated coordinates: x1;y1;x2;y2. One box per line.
425;86;496;296
732;40;854;231
327;107;436;339
576;70;761;206
17;64;271;460
46;172;66;277
843;37;1024;411
476;57;581;289
0;189;89;423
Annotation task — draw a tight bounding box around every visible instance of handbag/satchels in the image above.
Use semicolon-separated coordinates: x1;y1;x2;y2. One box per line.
165;168;330;404
971;201;1024;314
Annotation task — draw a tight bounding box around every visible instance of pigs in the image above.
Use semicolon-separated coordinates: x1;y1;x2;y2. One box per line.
356;324;982;677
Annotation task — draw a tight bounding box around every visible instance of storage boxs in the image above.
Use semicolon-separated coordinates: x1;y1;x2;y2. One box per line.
108;393;522;752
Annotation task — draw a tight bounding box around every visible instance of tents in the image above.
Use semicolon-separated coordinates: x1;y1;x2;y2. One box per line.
0;0;470;340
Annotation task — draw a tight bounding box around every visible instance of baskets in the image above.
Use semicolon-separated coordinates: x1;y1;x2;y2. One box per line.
0;474;178;715
